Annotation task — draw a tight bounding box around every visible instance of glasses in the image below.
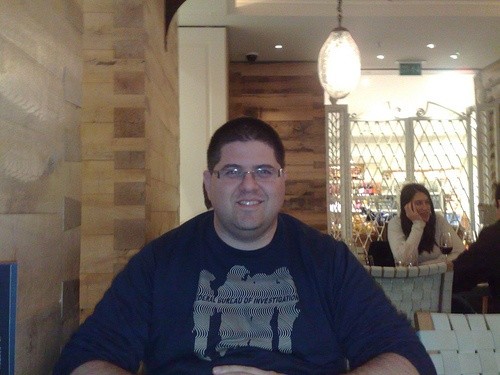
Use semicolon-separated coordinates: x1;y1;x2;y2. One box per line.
212;164;282;181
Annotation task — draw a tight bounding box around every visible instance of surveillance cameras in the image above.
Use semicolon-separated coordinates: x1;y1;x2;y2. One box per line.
245;52;258;65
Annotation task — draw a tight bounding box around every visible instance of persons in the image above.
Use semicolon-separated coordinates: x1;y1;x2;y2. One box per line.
453;184;500;314
53;118;438;375
387;183;467;265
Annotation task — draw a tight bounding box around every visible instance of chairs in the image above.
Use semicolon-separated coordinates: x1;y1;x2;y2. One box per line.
364;262;454;329
413;310;500;375
369;241;396;268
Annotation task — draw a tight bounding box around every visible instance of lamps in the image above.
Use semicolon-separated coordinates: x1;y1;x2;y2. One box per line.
316;0;360;100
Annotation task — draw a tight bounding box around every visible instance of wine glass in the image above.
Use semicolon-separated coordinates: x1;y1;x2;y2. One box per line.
440;231;453;262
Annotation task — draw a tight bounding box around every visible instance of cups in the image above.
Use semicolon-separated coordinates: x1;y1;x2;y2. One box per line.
409;253;418;267
462;230;478;250
394;258;406;267
347;240;354;251
